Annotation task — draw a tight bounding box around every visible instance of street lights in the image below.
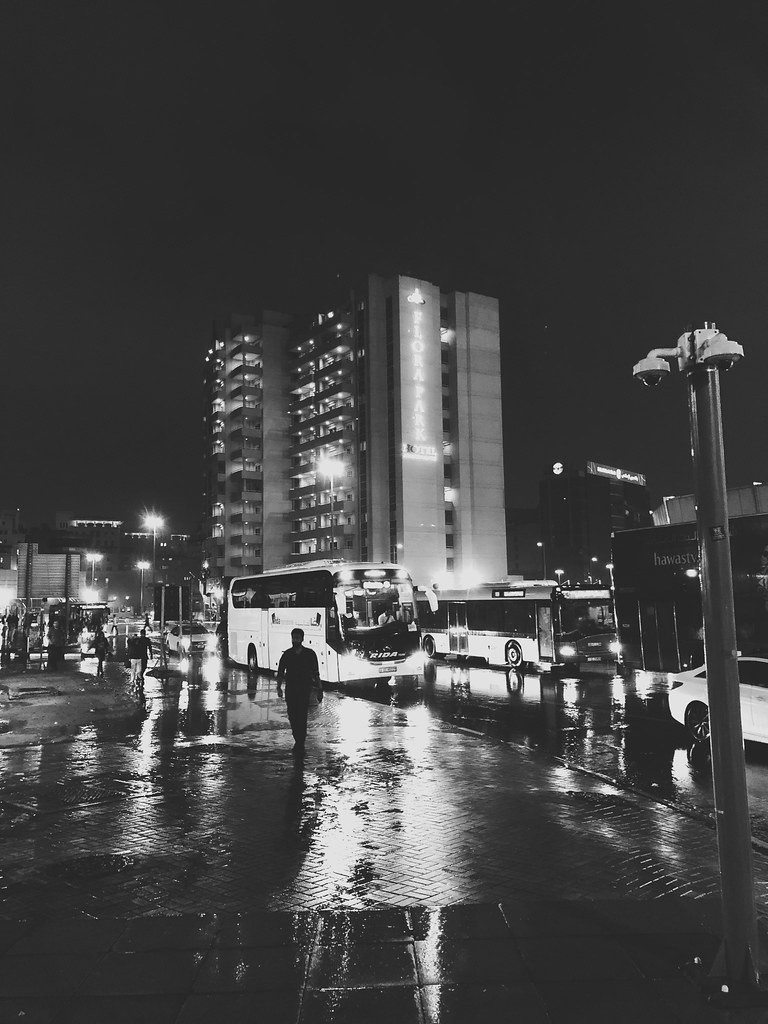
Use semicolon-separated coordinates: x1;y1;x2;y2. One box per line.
633;319;768;1008
554;569;564;584
146;513;165;622
535;540;547;580
136;561;150;614
86;552;103;606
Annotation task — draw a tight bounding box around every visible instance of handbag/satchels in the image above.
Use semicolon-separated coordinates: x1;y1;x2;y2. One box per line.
123;661;131;668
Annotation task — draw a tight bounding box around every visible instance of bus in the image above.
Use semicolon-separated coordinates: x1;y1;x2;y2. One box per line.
415;575;619;669
226;557;440;685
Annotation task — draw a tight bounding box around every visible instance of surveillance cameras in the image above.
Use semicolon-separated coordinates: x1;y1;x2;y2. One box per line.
633;357;670;387
704;341;744;371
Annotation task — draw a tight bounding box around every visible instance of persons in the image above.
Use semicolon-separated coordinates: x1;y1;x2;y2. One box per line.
109;613;119;635
87;631;114;676
2;608;34;644
378;608;396;627
277;629;323;752
128;630;154;688
47;619;64;669
143;613;154;633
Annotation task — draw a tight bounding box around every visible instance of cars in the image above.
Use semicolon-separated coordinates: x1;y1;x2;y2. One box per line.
163;622;218;656
665;655;767;744
78;611;166;654
